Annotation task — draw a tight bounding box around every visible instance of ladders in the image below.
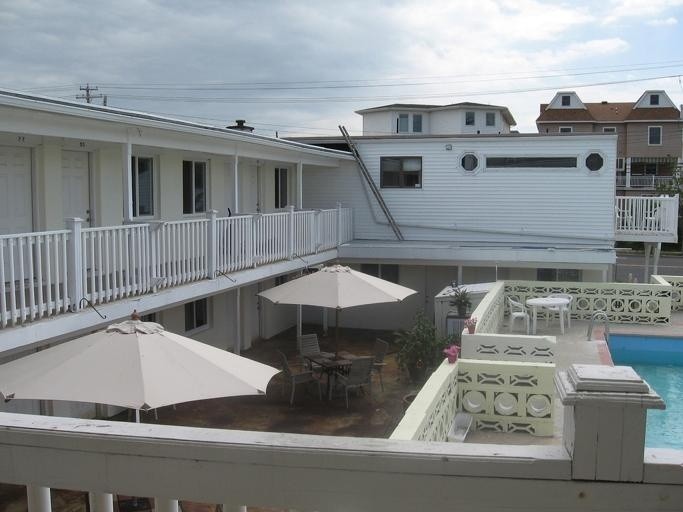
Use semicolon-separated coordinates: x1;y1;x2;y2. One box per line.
339;125;405;240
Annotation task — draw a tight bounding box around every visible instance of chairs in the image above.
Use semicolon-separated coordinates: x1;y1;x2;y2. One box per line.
614;207;665;231
507;295;529;334
270;334;322;405
545;293;573;328
328;338;389;408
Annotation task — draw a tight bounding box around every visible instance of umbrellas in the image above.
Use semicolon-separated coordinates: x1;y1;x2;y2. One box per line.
253;257;418;357
0;311;282;423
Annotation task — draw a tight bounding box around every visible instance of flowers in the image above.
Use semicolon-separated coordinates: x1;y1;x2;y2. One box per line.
464;317;477;328
443;344;459;356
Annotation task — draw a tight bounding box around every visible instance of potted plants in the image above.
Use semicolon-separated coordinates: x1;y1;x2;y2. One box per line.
394;309;439;384
447;288;472;316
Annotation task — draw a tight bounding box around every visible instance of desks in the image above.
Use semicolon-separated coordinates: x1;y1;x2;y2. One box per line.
525;298;569;335
301;350;358;399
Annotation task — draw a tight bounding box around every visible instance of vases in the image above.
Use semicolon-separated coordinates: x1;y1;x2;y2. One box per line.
447;354;456;363
467;326;475;334
